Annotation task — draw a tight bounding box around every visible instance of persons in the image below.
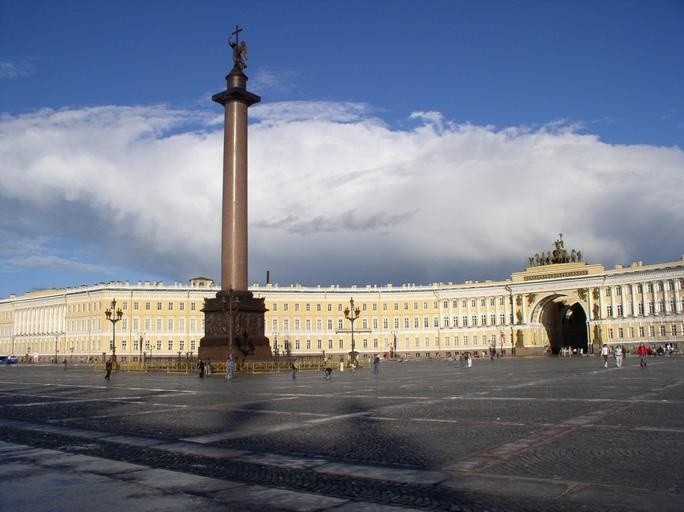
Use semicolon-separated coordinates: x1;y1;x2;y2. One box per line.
447;352;472;368
104;358;113;381
224;354;234;386
557;342;675;368
7;354;11;367
291;358;298;380
228;36;249;70
489;348;494;362
196;358;206;377
323;351;383;380
64;356;68;368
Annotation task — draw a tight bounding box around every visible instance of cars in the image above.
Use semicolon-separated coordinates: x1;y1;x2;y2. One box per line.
560;346;578;357
2;355;18;364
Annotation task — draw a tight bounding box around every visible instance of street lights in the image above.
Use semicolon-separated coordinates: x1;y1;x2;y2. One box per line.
51;331;64;363
390;330;398;358
219;284;240;361
23;346;32;364
272;331;292;361
321;349;327;367
102;297;123;372
343;297;360;366
177;350;193;372
136;331;155;371
69;347;75;365
